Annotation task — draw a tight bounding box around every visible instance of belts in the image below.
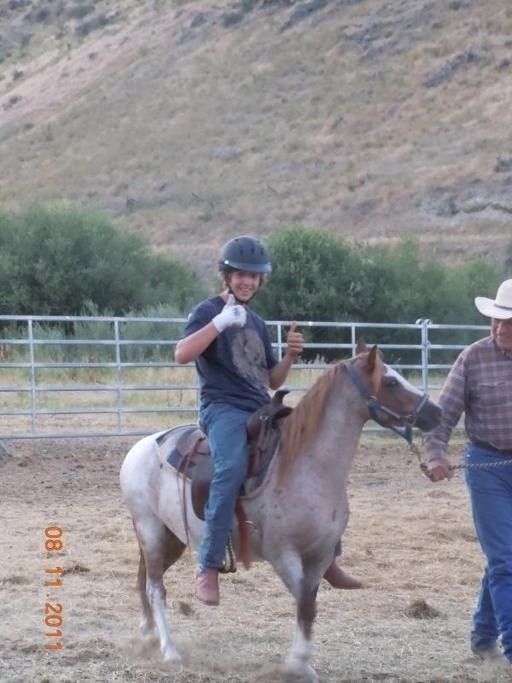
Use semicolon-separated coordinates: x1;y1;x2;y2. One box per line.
468;437;512;453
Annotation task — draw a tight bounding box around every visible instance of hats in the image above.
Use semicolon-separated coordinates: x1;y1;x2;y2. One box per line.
474;278;512;321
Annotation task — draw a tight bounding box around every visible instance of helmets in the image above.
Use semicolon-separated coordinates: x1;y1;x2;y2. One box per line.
218;235;272;274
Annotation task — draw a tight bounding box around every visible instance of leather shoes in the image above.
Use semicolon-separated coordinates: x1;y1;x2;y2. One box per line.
322;558;363;589
194;566;219;605
471;640;508;665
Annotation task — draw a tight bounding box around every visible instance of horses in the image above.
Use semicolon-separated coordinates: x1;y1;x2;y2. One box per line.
116;333;445;680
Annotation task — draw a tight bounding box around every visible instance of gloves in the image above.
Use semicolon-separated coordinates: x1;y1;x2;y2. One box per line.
212;294;247;332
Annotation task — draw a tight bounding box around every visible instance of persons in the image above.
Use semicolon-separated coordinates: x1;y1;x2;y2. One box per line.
177;234;362;603
420;277;511;662
